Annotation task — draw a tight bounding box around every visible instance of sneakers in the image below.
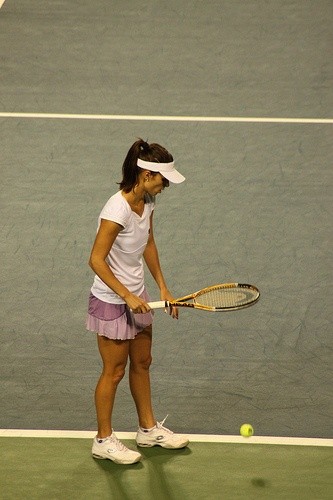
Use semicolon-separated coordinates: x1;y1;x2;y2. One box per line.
92;428;142;464
136;414;189;449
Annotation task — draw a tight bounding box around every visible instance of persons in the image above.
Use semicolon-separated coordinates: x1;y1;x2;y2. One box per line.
85;136;190;465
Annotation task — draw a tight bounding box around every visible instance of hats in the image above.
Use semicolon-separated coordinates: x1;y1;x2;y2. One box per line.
137;157;185;184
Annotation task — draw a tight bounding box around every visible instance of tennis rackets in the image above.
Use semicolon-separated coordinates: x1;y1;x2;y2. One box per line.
130;283;261;313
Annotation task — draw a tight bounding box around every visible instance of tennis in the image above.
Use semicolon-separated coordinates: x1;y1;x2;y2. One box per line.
239;424;253;438
